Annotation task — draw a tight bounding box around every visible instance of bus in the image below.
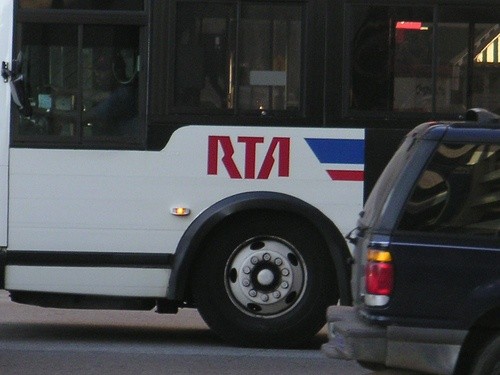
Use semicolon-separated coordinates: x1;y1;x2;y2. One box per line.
1;0;500;351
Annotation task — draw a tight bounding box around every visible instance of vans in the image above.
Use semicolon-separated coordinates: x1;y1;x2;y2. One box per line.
318;107;500;375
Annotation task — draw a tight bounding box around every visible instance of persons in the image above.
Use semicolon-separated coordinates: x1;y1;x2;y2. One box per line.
22;51;138;136
